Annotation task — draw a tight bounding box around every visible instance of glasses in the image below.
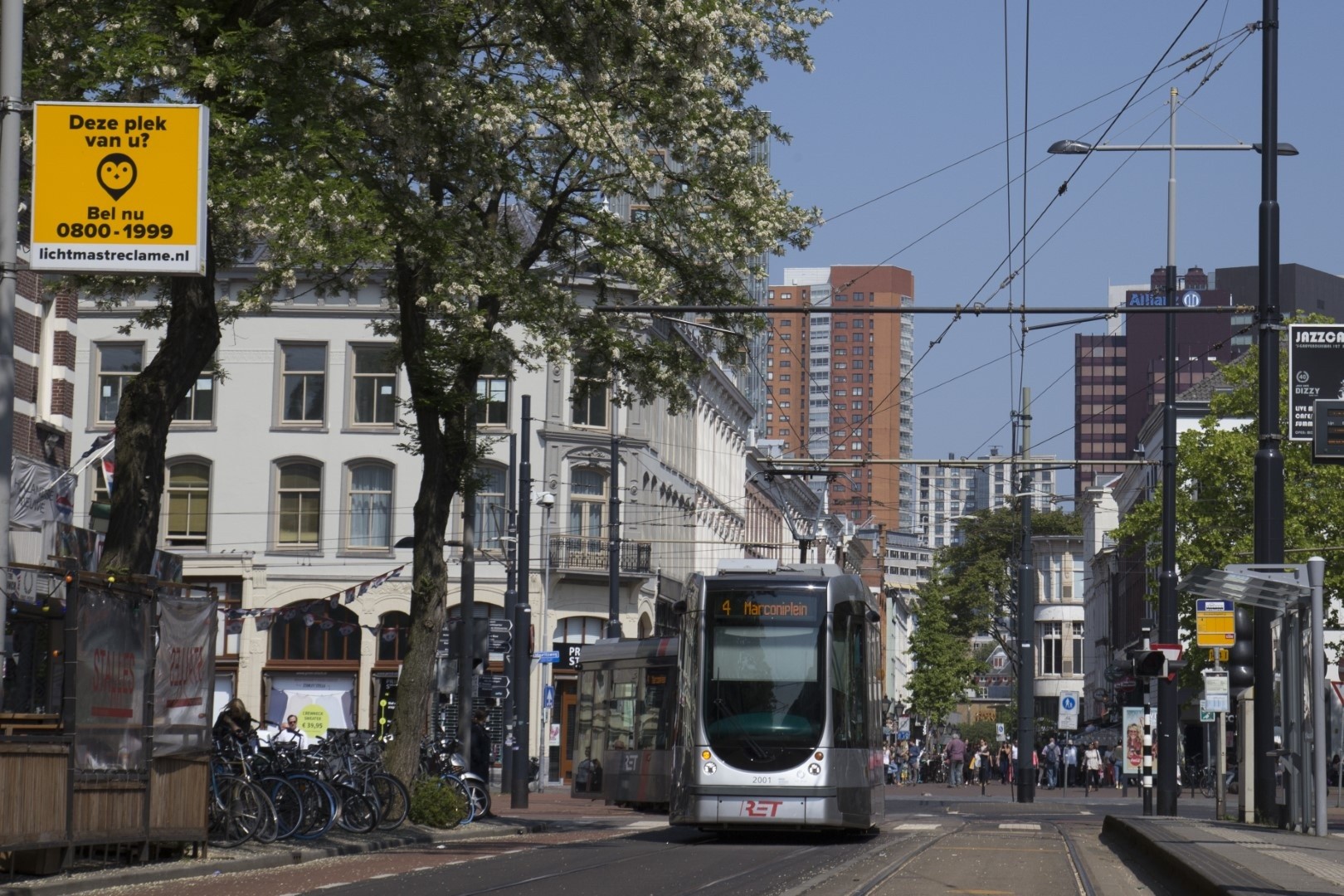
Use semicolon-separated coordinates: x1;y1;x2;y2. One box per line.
289;720;297;725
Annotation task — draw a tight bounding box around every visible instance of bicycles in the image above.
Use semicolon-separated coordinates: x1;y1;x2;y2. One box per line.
1180;753;1217;798
920;752;949;784
206;719;491;849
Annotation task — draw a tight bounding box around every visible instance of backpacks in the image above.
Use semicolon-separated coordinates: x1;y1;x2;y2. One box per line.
1046;745;1058;764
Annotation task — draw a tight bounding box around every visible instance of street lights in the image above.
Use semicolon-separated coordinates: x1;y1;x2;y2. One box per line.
881;693;892;742
534;494;555;792
1047;85;1301;816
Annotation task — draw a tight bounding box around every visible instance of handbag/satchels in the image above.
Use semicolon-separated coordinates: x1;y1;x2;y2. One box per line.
1109;748;1117;766
968;757;975;769
975;751;981;767
1098;768;1104;779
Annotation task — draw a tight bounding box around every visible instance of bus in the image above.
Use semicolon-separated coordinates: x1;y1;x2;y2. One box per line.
568;471;880;842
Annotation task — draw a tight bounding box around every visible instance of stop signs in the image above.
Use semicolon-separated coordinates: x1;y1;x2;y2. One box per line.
1114;679;1125;694
1120;676;1136;692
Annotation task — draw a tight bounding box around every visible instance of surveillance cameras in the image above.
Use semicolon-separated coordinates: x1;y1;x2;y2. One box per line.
542;494;554;506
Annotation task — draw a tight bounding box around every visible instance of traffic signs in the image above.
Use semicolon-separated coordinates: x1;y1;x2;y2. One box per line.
438;618;513;654
478;676;510;699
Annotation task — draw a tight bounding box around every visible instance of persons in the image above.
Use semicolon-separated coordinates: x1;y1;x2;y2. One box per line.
1126;723;1144;760
210;698;251;743
274;715;309;749
575;741;623;793
469;706;501;819
883;731;1124;792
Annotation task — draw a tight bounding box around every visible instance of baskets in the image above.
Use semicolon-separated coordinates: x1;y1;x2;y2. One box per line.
274;740;297;765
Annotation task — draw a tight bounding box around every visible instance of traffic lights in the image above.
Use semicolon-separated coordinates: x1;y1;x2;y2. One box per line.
1133;650;1166;676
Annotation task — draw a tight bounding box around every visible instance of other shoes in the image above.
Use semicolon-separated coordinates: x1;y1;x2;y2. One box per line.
484;811;497;818
